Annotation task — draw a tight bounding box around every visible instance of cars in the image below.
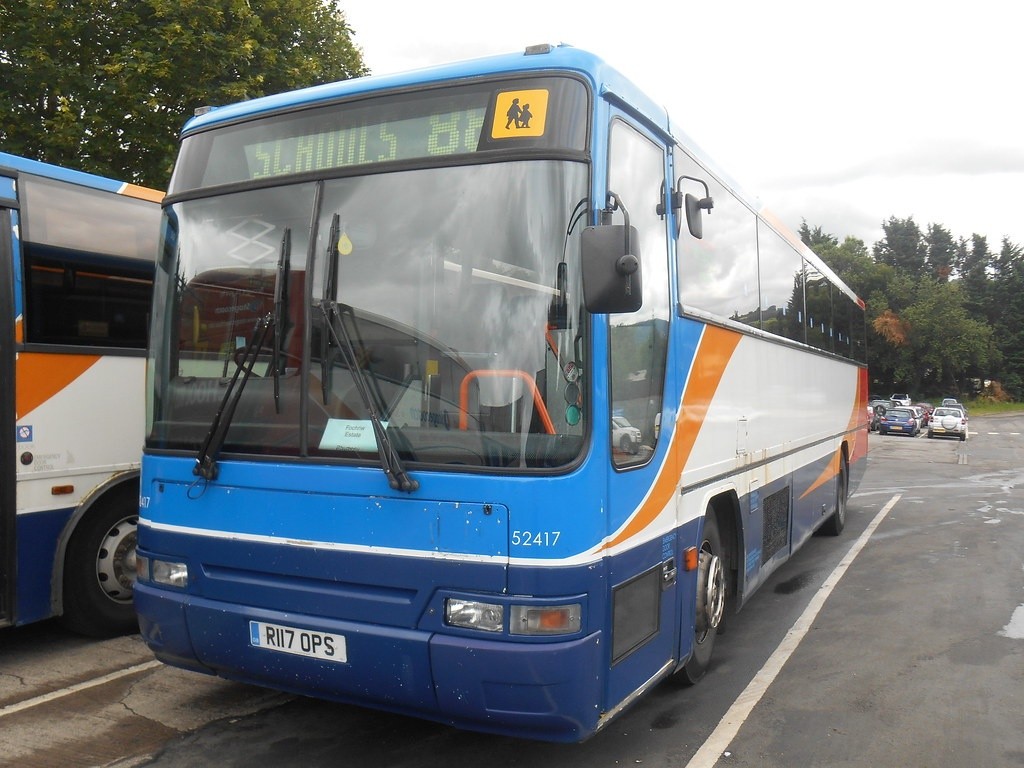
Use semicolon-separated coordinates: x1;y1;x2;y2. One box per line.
868;392;934;437
943;403;969;421
941;398;957;409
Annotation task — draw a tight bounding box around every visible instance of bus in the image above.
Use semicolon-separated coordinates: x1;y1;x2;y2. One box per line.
136;41;873;741
0;150;570;641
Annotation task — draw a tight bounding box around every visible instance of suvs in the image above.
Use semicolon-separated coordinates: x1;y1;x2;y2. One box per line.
927;407;968;441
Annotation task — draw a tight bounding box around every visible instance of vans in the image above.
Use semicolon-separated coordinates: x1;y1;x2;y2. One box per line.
611;416;642;455
